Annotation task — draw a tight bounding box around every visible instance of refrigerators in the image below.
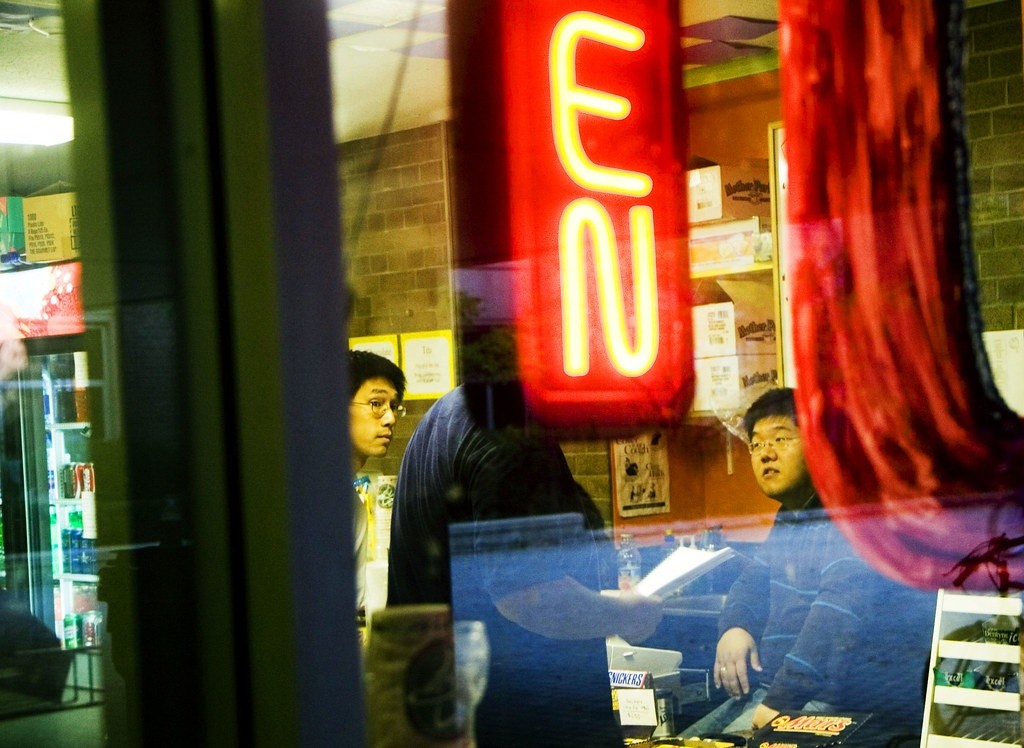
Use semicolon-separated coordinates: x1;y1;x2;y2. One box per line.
0;320;111;693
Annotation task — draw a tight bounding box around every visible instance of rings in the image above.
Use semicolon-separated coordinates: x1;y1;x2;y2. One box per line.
720;667;726;672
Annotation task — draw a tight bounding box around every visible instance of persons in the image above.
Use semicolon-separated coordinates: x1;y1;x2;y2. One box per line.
346;351;405;634
676;387;937;739
385;381;665;748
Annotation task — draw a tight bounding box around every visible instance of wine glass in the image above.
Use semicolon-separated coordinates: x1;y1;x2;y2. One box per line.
454;622;489;748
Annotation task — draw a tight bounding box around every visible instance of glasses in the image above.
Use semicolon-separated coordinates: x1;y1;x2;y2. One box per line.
350;400;408;418
748;435;801;454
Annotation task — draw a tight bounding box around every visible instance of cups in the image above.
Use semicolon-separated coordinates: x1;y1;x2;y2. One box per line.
359;475;400;646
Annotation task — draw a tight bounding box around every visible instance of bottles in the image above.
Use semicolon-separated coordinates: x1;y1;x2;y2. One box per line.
651;689;677;739
664;529;682;600
617;534;642;591
708;524;724;593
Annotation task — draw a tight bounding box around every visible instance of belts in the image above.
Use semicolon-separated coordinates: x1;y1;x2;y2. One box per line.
355;611;367;627
